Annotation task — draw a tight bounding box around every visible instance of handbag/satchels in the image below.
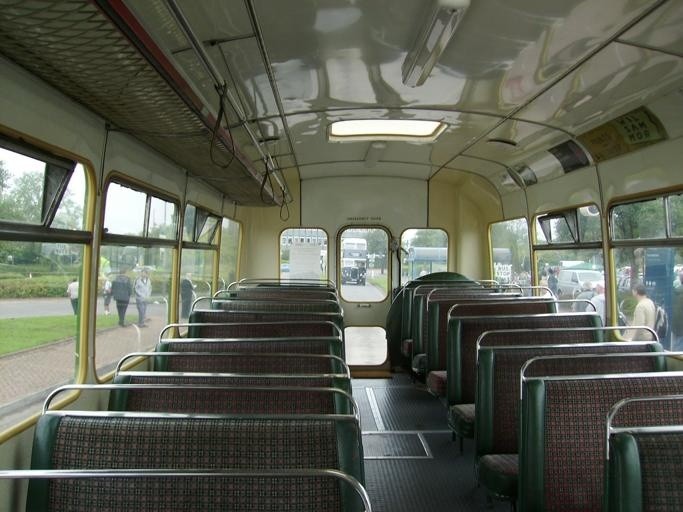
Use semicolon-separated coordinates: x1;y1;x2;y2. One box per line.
619;312;627;335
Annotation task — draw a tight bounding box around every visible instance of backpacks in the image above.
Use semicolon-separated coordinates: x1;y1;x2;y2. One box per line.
654;306;669;339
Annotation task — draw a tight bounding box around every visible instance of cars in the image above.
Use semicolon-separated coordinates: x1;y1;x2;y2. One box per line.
618;273;657;301
280;263;289;272
134;266;155;272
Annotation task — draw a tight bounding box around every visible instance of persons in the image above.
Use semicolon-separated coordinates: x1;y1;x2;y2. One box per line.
133;269;153;328
679;268;683;285
573;280;656;342
67;278;78;315
181;277;197;320
111;269;132;327
512;267;558;298
7;253;13;264
103;272;113;315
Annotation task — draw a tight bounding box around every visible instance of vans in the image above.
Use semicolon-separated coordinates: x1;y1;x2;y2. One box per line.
556;270;605;299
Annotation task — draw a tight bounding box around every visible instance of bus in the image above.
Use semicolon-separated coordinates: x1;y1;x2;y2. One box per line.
316;237;369;288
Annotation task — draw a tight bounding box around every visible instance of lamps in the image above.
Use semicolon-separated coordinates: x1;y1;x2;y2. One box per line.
363;141;386;168
402;0;471;87
